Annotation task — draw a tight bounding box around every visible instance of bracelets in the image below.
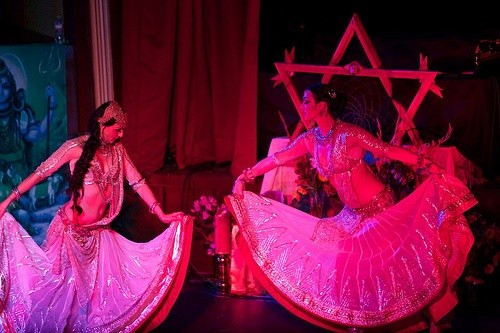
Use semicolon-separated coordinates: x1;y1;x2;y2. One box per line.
149;202;159;214
12;189;20;201
243;167;255;183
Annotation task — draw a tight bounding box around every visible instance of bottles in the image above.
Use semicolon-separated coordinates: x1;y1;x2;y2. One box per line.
54;16;66;45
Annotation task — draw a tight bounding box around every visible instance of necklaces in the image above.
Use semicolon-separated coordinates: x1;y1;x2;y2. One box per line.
97;148;111;155
312;121;339;179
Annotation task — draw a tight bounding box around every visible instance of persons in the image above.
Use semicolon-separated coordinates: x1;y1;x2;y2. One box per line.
0;101;196;333
223;84;478;333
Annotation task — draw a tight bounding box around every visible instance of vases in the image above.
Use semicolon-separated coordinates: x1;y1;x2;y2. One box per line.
213;253;231;295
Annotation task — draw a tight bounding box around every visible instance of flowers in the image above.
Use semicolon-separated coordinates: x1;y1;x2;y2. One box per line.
292;162;336;214
382;161;415;195
190;195;217;256
460;209;500;286
